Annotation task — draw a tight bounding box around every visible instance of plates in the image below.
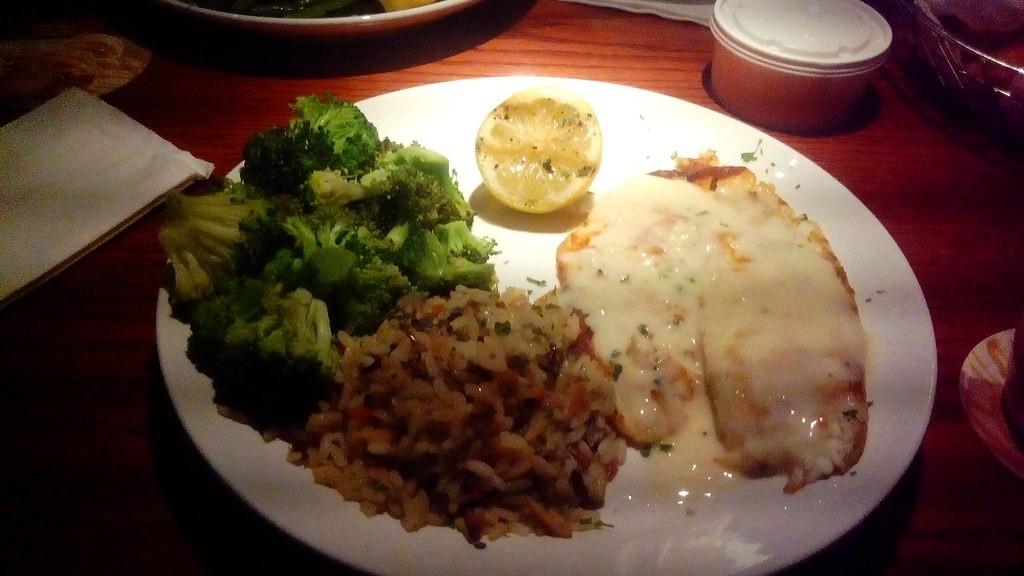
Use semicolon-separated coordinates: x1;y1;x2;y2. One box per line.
160;0;476;37
156;75;937;576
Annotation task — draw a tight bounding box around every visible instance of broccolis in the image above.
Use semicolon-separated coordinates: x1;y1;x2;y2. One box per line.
157;89;497;433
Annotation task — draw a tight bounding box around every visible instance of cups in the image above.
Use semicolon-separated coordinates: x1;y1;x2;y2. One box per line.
1003;327;1023;453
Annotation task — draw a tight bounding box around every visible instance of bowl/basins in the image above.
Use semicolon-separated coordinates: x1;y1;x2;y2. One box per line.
906;0;1024;132
708;0;894;134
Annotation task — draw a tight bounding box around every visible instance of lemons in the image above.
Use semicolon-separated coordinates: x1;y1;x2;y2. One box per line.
475;84;602;213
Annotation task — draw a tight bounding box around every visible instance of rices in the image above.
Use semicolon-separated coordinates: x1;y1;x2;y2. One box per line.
221;288;630;550
